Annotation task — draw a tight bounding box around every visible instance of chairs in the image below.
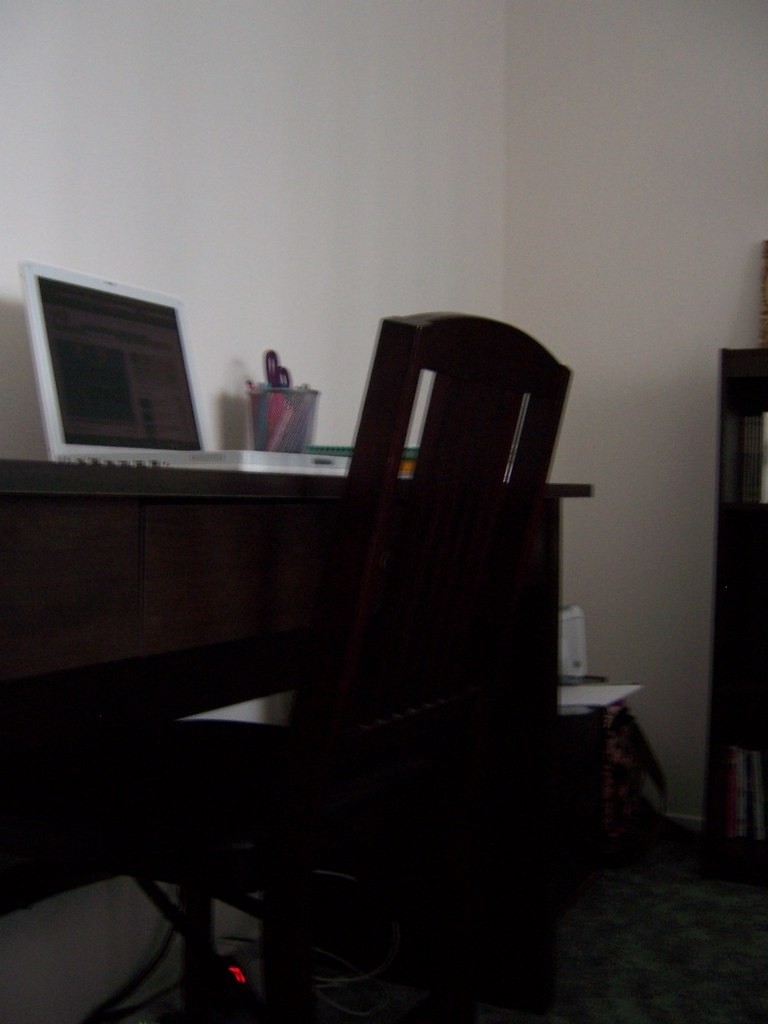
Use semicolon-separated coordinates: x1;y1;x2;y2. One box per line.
171;315;571;1024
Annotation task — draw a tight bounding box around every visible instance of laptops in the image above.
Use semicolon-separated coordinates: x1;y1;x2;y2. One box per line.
17;260;351;478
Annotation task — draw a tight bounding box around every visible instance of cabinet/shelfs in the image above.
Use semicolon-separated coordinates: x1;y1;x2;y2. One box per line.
697;348;768;890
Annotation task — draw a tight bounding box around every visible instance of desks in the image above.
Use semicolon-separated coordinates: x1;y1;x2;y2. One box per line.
2;464;590;965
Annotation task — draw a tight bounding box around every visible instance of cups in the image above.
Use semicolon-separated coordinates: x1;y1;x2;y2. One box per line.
245;384;319;451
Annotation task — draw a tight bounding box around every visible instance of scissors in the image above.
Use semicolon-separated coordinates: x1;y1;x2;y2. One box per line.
263;347;293;390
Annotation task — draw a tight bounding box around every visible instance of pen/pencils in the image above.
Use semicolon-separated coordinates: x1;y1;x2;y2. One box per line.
244;378;320;454
